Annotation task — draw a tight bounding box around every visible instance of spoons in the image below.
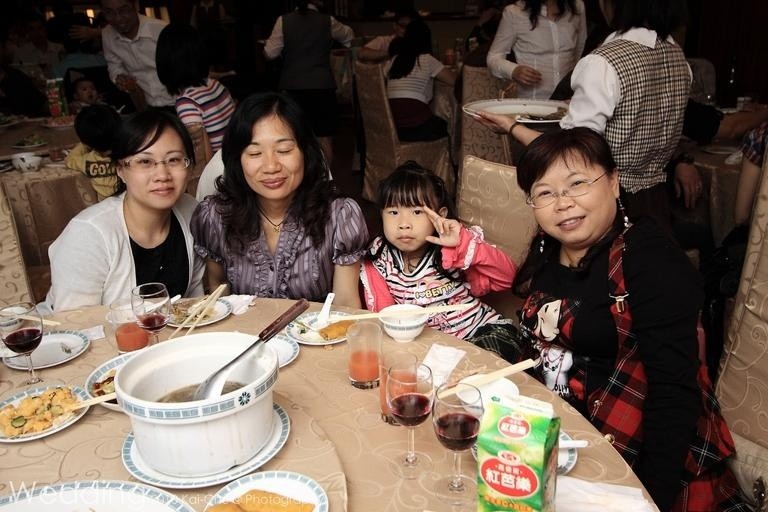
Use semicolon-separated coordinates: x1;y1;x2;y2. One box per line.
311;288;334;335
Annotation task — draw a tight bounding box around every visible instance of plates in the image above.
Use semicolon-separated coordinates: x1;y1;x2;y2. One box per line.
116;400;297;490
0;479;195;512
266;333;300;369
165;295;238;330
0;384;92;443
705;145;740;156
283;311;360;346
36;117;76;130
9;138;47;148
469;418;591;480
85;349;144;411
460;95;571;123
2;328;91;371
196;472;328;512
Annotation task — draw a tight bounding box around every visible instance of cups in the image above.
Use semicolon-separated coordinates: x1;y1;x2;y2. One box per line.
377;350;420;428
106;298;149;354
346;321;387;390
47;142;68;161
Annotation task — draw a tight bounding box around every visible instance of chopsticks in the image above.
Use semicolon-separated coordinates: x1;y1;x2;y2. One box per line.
0;310;61;329
424;355;535;394
170;279;230;337
333;302;481;323
59;392;118;413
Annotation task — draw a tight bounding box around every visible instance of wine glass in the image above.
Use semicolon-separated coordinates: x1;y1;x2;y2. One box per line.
388;361;434;482
431;379;491;500
0;300;44;386
131;281;172;347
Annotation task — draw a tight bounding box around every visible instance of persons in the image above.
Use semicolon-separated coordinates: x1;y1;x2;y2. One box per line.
26;105;209;319
188;88;370;310
548;19;768;211
466;0;695;255
701;116;768;385
264;1;354;168
354;157;547;386
655;10;717;106
504;125;743;512
485;0;587;101
0;1;236;165
361;7;457;142
454;1;502;105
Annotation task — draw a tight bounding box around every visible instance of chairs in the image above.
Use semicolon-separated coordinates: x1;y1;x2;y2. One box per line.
0;49;768;510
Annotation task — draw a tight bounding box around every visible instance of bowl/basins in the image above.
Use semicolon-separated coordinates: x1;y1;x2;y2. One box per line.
0;305;26;332
106;300;154;327
8;152;35;169
17;157;42;172
455;373;520;418
378;302;428;347
113;332;279;478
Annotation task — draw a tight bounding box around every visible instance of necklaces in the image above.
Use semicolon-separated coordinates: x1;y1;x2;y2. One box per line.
255;204;294;233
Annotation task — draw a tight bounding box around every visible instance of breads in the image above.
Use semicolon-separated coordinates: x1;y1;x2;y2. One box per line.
206;490;315;512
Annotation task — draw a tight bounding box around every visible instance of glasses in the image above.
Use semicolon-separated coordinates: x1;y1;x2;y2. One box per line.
114;153;192;171
526;169;609;209
